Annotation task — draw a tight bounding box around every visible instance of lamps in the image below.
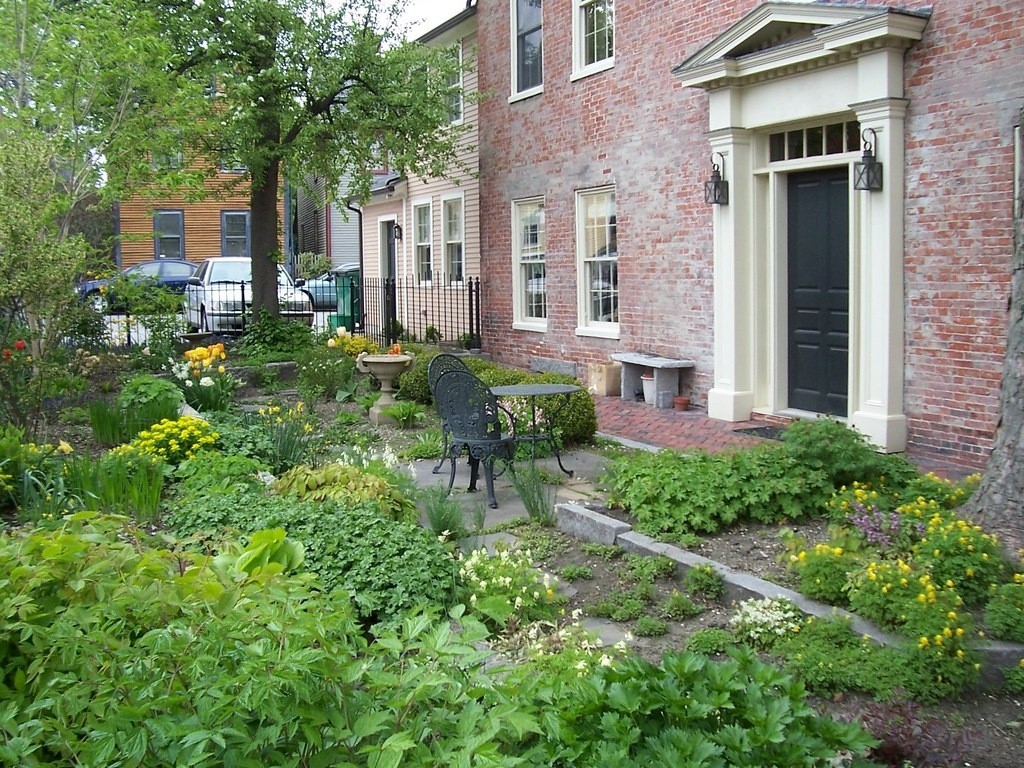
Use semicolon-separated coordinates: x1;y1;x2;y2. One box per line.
853;127;884;192
704;151;730;205
393;222;402;240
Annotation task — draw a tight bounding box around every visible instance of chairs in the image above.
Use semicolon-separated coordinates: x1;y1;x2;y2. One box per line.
434;370;519;509
429;353;498;481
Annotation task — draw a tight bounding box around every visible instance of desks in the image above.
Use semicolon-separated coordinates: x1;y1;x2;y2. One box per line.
181;332;213;349
488;384;581;480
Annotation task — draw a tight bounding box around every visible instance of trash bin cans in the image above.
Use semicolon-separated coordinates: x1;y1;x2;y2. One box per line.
328;264;363;322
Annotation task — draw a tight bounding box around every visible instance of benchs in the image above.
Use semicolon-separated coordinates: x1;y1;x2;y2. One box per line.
613;352;695;406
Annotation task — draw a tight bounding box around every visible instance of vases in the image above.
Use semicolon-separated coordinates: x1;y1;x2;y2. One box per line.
356;350;416;405
640;375;654;405
672;397;691;411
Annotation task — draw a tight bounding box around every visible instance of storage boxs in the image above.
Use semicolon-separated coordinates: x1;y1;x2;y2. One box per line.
587;361;622;396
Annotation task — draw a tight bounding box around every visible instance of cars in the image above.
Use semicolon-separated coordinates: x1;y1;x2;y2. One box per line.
72;260;198;315
296;263;360;309
183;256;315;333
